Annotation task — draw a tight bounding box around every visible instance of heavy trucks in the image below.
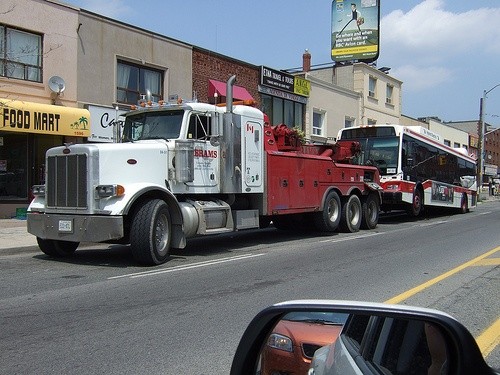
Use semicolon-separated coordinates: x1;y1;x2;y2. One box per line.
27;75;381;265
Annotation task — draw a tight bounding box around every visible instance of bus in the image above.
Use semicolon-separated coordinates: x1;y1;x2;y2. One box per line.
336;125;478;217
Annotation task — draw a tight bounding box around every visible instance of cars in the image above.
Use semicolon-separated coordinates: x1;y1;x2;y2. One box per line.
261;311;350;375
